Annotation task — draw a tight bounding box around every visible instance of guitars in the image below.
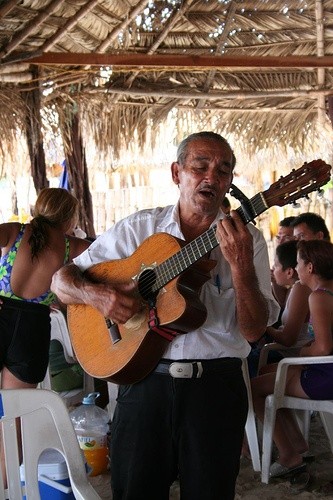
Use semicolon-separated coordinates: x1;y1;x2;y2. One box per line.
63;158;332;385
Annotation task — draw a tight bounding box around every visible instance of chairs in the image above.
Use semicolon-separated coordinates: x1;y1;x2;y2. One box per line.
0;309;333;500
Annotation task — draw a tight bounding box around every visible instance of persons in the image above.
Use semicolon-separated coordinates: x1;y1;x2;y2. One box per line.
254;238;315;439
0;188;94;390
290;212;332;242
249;239;333;476
50;131;282;500
276;216;298;246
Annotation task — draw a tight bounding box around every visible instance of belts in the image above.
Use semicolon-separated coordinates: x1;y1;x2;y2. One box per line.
152;357;242;379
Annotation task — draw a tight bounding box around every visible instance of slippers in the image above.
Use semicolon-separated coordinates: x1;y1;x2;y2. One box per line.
270;460;306;477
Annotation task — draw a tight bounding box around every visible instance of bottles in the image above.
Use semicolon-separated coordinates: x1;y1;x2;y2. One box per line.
69;392;111;477
